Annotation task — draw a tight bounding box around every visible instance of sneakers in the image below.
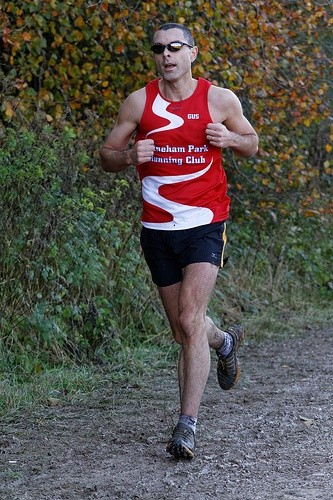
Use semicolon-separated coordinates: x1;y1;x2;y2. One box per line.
166;423;195;458
217;323;245;391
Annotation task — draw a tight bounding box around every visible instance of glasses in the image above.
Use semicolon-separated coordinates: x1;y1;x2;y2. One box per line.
149;40;192;54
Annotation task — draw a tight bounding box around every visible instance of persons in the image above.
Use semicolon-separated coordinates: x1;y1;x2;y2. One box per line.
100;26;258;459
